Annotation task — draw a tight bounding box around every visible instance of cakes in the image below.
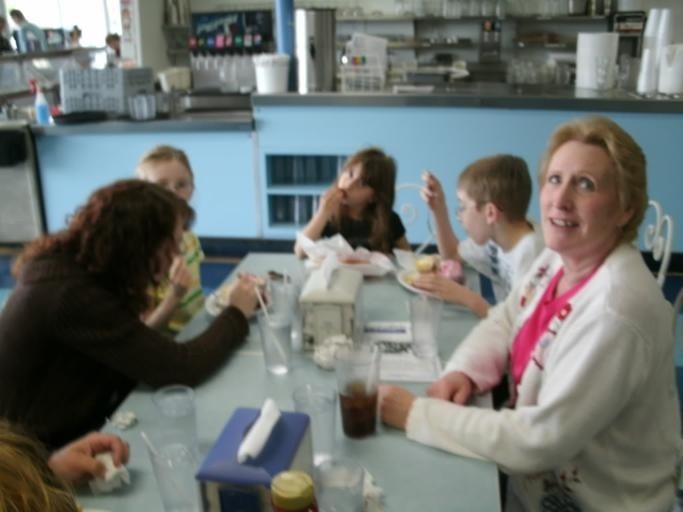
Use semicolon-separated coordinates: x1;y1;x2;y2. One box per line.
439;259;464;284
414;255;435;276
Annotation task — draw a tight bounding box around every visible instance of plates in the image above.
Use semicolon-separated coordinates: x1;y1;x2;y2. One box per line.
305;259;386;280
205;285;221;318
398;267;431;298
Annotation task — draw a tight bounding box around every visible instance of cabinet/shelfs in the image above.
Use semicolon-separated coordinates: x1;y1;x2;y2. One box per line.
2;44;108;102
335;5;611;87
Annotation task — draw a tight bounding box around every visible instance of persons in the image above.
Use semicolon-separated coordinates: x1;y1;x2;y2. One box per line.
0;180;265;456
0;422;130;512
412;155;546;320
137;146;208;344
0;9;121;67
294;147;411;260
377;115;683;512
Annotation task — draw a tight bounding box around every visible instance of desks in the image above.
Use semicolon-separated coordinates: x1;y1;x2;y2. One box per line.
74;246;506;506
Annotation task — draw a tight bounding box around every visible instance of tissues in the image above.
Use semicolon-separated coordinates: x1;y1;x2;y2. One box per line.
299;248;365;352
195;397;316;511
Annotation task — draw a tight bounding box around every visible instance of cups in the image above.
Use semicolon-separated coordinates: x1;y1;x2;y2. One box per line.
148;386;203;512
256;278;304;377
128;94;156;120
635;7;673;96
407;293;444;358
293;342;382;511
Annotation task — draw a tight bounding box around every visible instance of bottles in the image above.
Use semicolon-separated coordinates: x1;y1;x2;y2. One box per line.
272;472;316;512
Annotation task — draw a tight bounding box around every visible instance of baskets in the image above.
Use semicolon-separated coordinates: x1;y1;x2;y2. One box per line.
59;65;155;117
335;65;388;94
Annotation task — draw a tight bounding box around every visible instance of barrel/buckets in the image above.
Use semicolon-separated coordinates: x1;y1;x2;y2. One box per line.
252;52;290;94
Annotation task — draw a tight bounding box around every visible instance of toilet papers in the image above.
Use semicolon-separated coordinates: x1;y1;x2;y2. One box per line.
575;31;620;96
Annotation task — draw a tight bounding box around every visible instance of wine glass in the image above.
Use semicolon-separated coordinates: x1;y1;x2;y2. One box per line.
594;53;612;97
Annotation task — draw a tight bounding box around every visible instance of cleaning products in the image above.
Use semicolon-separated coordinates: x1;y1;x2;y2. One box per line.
25;72;50;124
